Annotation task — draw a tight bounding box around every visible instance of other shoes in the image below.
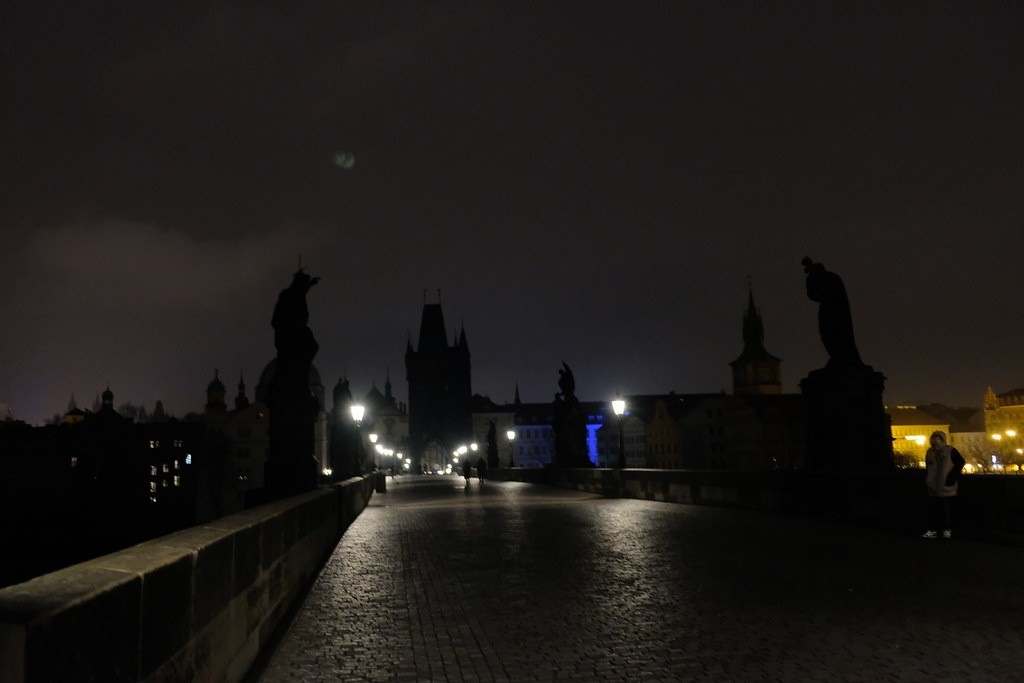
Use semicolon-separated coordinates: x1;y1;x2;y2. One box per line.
943;529;951;538
922;530;937;539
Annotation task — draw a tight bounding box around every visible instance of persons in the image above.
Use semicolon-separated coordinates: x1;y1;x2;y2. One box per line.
477;455;486;485
418;463;422;474
423;463;428;474
922;430;966;539
463;456;472;488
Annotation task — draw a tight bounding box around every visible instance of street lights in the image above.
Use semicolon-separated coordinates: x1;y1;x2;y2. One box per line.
349;402;367;477
505;427;517;467
368;431;379;472
610;390;628;468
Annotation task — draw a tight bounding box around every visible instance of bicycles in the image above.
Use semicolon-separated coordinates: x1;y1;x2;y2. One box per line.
463;468;471;487
478;471;489;487
